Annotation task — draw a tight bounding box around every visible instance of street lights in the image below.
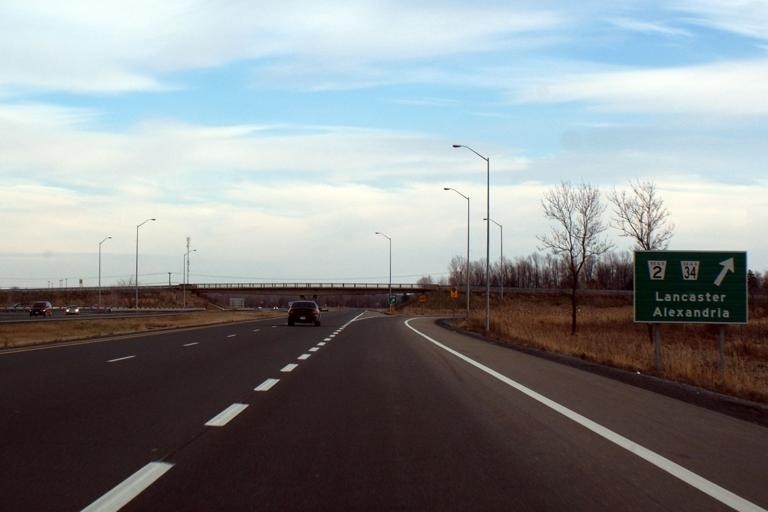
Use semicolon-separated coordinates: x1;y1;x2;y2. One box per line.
184;250;196;308
99;236;112;304
375;232;392;312
136;219;156;309
444;145;504;331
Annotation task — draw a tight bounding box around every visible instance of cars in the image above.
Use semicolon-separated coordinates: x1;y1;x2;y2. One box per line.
287;300;322;326
10;300;53;318
64;304;81;315
